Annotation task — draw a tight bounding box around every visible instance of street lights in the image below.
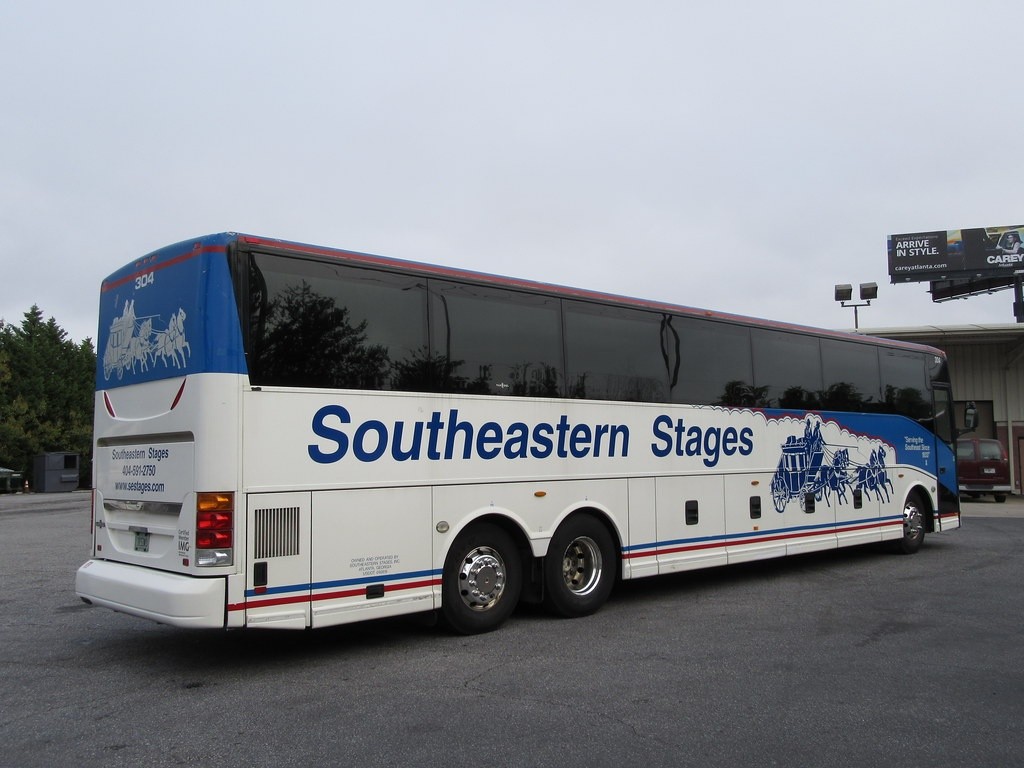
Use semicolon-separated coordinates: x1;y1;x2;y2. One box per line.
834;282;878;334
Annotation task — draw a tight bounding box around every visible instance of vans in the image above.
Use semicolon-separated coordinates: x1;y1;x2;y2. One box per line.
948;438;1013;502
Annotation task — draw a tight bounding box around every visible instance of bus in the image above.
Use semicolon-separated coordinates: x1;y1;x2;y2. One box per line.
70;231;963;642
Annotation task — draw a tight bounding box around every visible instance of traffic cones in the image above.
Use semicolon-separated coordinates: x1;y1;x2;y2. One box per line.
23;479;30;493
